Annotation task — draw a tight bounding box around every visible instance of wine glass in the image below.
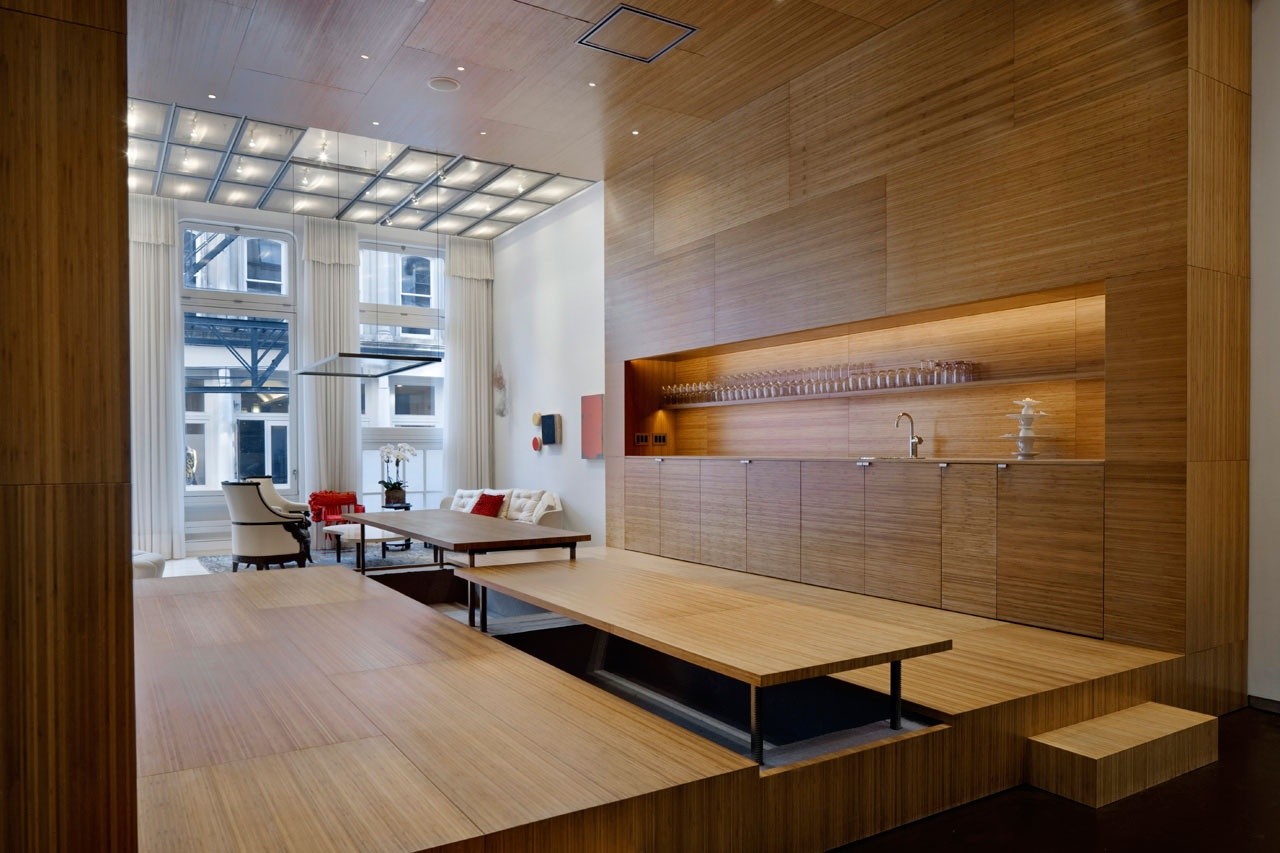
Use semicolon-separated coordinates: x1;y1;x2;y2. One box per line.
712;362;875;401
916;360;934;384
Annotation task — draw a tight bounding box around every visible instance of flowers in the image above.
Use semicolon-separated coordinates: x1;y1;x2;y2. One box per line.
378;442;417;491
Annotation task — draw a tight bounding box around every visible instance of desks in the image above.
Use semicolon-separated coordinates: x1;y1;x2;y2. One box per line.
453;563;952;766
344;510;593;629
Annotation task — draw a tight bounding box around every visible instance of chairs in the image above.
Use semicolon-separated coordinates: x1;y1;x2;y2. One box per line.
309;489;366;559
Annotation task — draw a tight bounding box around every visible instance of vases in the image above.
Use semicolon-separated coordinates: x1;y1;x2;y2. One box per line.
384;489;407;505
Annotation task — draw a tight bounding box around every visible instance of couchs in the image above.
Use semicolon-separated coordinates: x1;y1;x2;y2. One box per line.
221;480;310;572
244;475;314;564
439;493;563;530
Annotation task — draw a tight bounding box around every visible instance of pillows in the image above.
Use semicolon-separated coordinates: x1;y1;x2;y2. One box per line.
485;488;514;519
509;488;544;521
449;488;484;513
469;494;505;518
532;491;557;524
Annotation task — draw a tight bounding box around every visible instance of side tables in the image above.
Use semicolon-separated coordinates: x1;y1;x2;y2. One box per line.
382;505;414;549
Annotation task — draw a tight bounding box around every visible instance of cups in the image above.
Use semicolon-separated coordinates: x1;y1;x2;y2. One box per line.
1019;418;1034;436
1016;438;1035;453
1021;405;1034;414
877;367;916;388
662;381;715;403
934;360;974;384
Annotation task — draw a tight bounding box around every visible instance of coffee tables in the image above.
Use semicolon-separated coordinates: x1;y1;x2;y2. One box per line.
323;524;410;568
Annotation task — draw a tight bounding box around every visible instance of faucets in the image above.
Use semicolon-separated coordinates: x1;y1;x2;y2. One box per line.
894;412;923;458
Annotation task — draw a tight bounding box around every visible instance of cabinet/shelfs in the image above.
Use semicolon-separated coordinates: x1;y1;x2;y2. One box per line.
998;459;1107;639
799;458;942;611
699;457;802;585
937;460;996;621
622;455;701;562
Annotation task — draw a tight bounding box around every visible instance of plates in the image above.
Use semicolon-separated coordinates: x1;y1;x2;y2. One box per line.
1013;401;1041;405
1007;413;1051;419
1011;452;1041;461
1000;435;1055;438
